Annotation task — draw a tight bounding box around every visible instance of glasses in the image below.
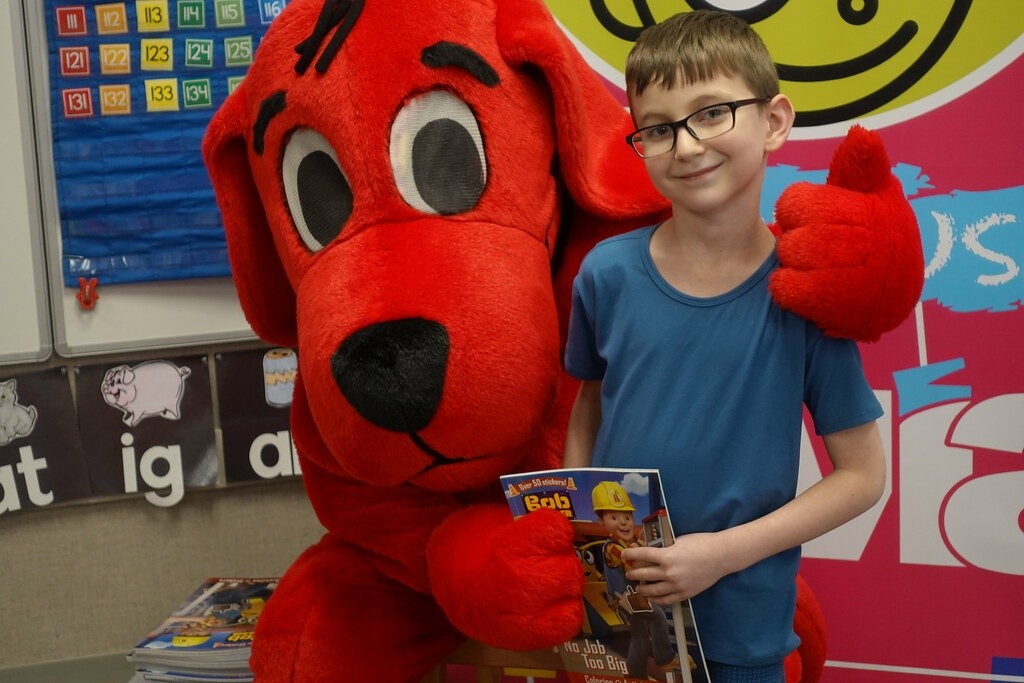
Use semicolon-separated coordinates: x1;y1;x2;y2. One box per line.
626;97;771;158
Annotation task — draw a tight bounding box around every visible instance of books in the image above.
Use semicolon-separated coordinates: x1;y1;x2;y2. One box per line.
126;576;282;683
500;464;712;683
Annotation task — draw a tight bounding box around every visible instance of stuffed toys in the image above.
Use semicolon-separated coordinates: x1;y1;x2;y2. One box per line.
199;0;923;683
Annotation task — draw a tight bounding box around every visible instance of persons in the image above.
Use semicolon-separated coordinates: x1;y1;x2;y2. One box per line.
565;11;887;683
